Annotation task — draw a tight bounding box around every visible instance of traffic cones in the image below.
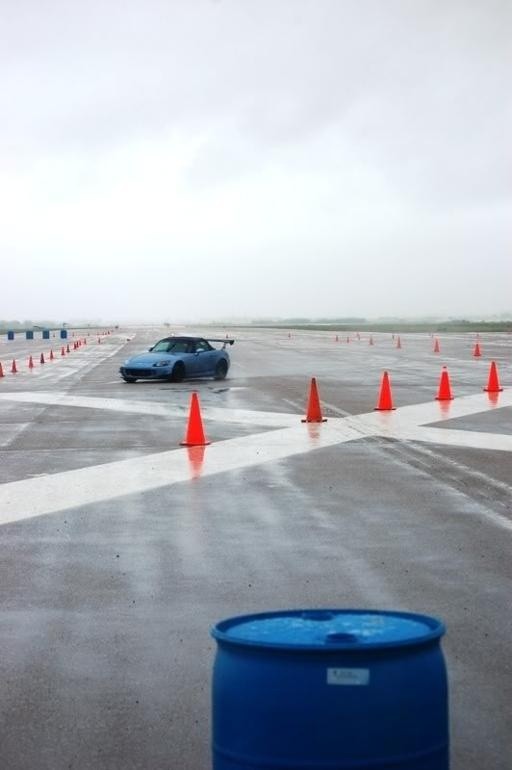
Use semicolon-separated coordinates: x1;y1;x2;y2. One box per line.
179;391;211;446
39;353;45;364
10;360;18;373
433;339;440;353
333;330;482;350
483;360;503;392
473;343;481;357
50;327;115;359
373;371;396;410
0;363;5;377
434;366;455;401
300;377;328;424
29;356;34;368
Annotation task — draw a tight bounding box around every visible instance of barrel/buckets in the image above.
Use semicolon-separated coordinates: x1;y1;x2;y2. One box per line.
208;608;449;770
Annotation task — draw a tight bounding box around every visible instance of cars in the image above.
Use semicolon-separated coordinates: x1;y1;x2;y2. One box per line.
120;335;235;383
